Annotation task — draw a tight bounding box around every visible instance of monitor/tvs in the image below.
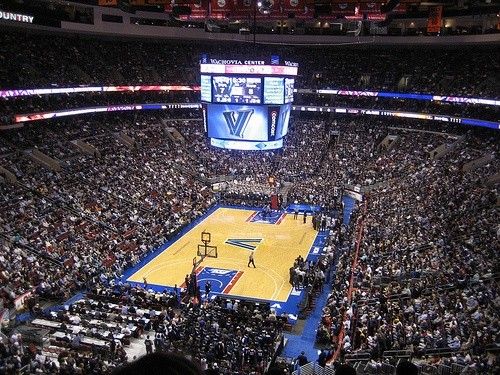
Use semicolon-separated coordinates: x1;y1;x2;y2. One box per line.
200;64;298;150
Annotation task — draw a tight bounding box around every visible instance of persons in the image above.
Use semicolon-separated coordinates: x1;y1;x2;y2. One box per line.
2;27;499;375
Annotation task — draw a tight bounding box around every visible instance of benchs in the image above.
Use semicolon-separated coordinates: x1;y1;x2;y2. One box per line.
0;0;500;375
